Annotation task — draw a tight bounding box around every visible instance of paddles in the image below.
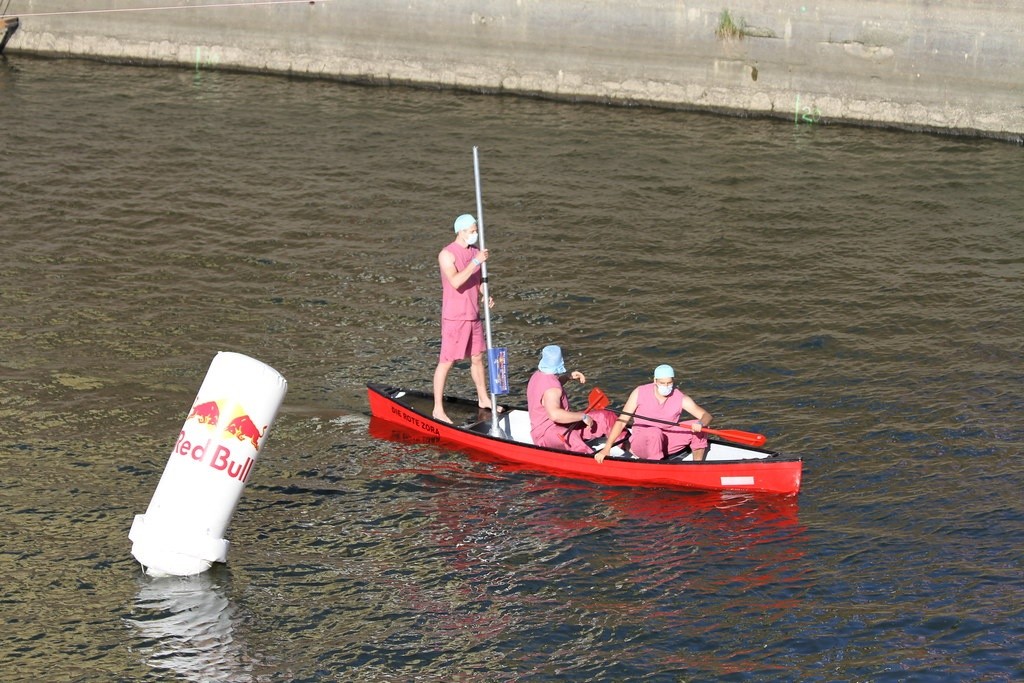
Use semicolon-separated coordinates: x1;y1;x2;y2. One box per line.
557;387;610;443
598;403;766;447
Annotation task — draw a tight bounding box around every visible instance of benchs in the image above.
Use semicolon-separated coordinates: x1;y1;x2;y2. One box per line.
588;436;626;453
624;448;692;460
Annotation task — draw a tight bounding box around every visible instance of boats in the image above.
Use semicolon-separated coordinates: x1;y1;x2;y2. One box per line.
365;381;803;497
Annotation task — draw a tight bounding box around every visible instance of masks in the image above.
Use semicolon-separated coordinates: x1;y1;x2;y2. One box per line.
462;232;478;244
655;383;673;396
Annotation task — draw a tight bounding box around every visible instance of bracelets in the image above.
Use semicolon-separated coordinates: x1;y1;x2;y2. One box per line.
472;259;479;265
697;422;703;426
583;414;586;420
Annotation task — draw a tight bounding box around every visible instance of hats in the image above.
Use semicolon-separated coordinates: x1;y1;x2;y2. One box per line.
538;345;566;374
654;364;674;379
454;214;476;233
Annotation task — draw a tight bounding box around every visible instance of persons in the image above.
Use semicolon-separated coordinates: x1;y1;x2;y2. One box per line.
594;364;712;463
433;214;505;424
527;346;632;455
